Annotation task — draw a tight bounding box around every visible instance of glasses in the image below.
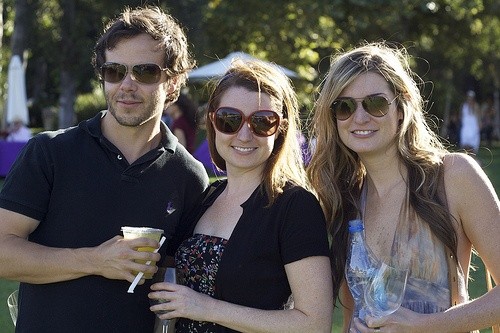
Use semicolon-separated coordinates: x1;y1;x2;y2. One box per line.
329;94;401;121
211;106;285;137
99;61;167;86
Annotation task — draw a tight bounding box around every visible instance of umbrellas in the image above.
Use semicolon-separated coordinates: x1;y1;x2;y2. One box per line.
187;52;298;82
6;54;29;125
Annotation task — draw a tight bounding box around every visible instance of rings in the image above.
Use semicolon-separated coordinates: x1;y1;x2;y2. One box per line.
373;328;380;333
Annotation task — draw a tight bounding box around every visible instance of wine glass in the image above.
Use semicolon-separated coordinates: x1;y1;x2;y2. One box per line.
150;268;176;333
364;258;408;318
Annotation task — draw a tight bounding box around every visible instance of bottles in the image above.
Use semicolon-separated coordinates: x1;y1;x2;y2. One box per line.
345;220;381;318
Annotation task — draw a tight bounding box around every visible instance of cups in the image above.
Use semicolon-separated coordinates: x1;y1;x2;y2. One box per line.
120;226;164;279
8;290;18;326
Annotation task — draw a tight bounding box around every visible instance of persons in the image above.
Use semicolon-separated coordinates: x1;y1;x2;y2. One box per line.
148;58;333;333
0;7;210;333
448;91;500;152
306;42;500;333
